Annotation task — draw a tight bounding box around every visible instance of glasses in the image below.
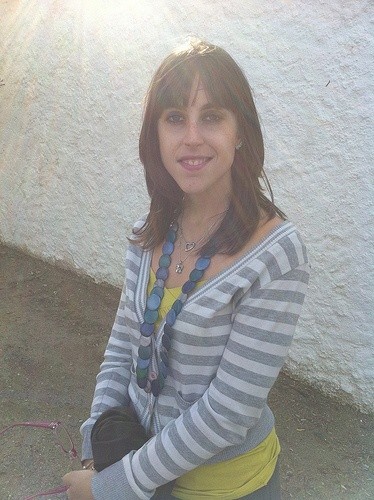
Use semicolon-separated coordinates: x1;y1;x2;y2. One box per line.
0;419;86;500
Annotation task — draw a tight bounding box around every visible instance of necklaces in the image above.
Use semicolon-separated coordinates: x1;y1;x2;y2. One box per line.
175;195;216;274
135;206;214;398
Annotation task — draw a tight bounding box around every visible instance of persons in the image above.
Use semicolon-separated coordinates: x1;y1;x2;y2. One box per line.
61;42;312;500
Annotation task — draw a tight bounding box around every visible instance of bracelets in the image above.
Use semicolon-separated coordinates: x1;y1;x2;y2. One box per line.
83;460;97;472
